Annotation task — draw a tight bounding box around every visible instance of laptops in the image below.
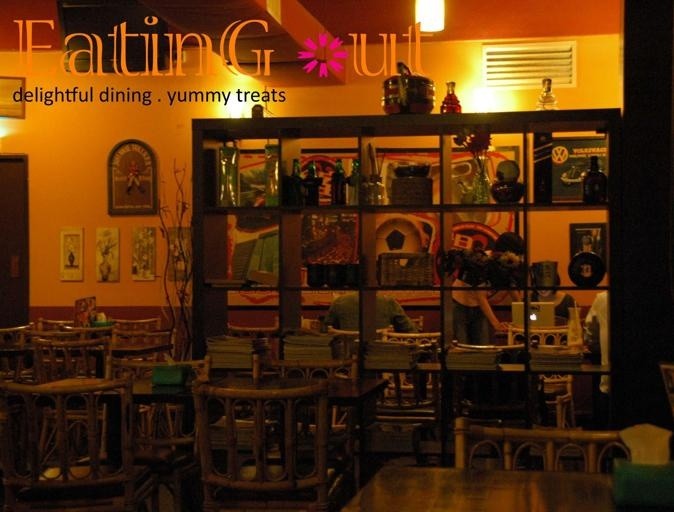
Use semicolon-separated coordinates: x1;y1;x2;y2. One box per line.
511;301;555;328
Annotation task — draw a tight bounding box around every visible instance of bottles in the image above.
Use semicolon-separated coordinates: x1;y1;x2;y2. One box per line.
567;305;584;346
330;159;347;206
536;78;557;110
349;158;360;205
440;81;462;114
303;159;320;206
580;155;607;203
287;158;303;206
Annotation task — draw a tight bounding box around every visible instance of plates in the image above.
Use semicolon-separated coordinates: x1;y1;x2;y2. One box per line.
568;252;605;288
93;320;113;326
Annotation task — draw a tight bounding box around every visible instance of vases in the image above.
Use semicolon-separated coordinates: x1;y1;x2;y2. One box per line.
168;314;192;362
469;156;493;206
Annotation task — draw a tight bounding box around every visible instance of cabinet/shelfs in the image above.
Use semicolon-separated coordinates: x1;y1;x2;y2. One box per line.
184;104;624;512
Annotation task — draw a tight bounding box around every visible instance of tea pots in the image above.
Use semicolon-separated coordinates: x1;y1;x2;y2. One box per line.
381;59;436;115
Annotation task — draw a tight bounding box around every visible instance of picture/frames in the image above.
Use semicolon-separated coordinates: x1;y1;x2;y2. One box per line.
58;225;85;281
105;137;160;217
567;221;612;276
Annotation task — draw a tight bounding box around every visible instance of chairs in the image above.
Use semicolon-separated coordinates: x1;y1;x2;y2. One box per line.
0;309;673;511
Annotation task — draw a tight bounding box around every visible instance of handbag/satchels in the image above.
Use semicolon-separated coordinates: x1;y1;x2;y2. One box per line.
152;366;187;386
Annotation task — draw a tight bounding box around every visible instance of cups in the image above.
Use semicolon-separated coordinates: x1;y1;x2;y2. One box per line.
324;263;346;289
344;264;364;288
528;260;557;287
304;262;325;286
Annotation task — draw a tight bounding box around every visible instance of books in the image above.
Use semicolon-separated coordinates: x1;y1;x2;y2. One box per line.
207;332;585;371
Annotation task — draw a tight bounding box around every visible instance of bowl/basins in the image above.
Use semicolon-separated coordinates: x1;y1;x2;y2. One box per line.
391;163;431;177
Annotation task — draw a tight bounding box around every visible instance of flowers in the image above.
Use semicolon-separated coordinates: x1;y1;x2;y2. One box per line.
449;124;492;172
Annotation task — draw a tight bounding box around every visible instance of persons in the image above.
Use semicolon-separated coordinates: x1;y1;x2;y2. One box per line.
452;232;524;343
326;289;420;340
529;272;576;321
588;290;610;400
